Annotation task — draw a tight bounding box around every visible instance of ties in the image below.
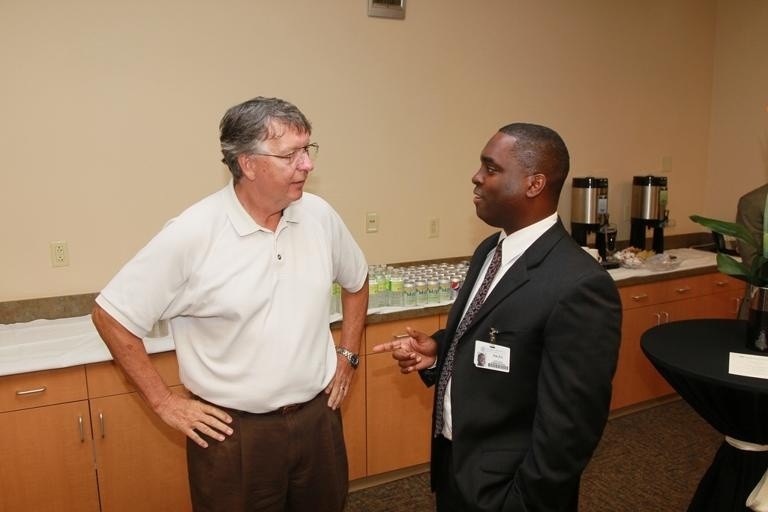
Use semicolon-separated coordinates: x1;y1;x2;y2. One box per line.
432;237;507;438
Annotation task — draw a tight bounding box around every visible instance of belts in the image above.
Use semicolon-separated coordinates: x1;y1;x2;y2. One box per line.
191;390;324;418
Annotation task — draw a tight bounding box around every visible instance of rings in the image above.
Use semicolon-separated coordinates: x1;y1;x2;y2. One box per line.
338;385;346;394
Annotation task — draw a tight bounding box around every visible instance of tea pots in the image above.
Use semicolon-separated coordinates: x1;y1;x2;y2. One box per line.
746;263;768;352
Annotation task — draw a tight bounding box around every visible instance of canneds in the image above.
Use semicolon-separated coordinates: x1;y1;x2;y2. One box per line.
395;260;471;306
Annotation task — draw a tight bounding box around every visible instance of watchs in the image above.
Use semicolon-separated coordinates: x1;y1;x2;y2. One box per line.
334;345;359;371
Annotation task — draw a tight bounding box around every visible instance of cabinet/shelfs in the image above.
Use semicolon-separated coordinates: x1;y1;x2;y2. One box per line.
1;343;194;512
327;315;368;497
711;261;752;324
366;308;440;487
603;266;712;423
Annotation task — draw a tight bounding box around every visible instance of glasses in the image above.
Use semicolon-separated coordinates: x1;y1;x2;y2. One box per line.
250;142;319;163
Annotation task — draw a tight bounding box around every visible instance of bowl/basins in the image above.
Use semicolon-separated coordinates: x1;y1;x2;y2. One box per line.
613;251;690;272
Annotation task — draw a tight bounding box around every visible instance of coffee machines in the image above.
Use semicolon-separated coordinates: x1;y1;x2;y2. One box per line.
570;176;620;270
631;174;669;261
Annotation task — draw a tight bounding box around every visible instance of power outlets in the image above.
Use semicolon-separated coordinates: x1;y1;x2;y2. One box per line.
50;240;70;269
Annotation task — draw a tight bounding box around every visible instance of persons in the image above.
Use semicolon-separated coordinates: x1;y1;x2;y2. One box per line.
373;121;622;512
91;96;373;512
734;180;767;287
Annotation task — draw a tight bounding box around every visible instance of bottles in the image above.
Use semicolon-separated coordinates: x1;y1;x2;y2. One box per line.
331;263;403;315
